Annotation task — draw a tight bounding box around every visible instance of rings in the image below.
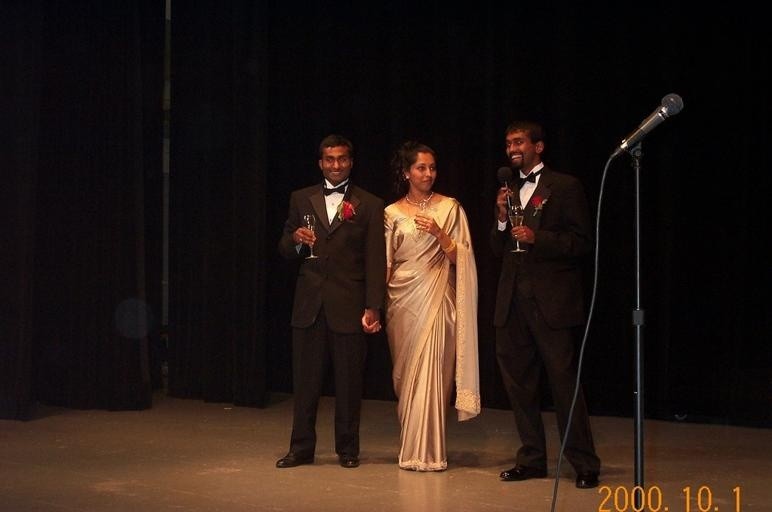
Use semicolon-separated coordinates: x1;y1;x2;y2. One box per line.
424;223;427;227
423;219;428;223
514;234;518;239
299;238;302;241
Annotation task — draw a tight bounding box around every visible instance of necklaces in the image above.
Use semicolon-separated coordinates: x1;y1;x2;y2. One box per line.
404;189;434;207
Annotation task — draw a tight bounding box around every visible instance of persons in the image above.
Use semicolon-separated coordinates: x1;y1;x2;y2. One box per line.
486;118;602;488
275;133;388;468
359;139;479;472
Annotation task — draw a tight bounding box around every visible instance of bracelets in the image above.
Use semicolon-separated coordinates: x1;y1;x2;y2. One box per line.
440;238;457;254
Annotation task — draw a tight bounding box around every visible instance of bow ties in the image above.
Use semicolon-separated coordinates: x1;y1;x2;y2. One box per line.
517;172;536;188
324;186;345;196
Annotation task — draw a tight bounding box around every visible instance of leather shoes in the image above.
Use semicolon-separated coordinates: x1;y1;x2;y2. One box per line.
500;464;548;480
576;469;601;488
339;451;359;468
276;450;314;467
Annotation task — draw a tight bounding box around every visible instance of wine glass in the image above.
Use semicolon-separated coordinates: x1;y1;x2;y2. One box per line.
507;204;527;254
302;213;319;261
416;201;428;239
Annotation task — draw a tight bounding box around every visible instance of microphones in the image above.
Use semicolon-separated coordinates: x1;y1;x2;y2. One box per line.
615;93;684;154
498;166;511;208
